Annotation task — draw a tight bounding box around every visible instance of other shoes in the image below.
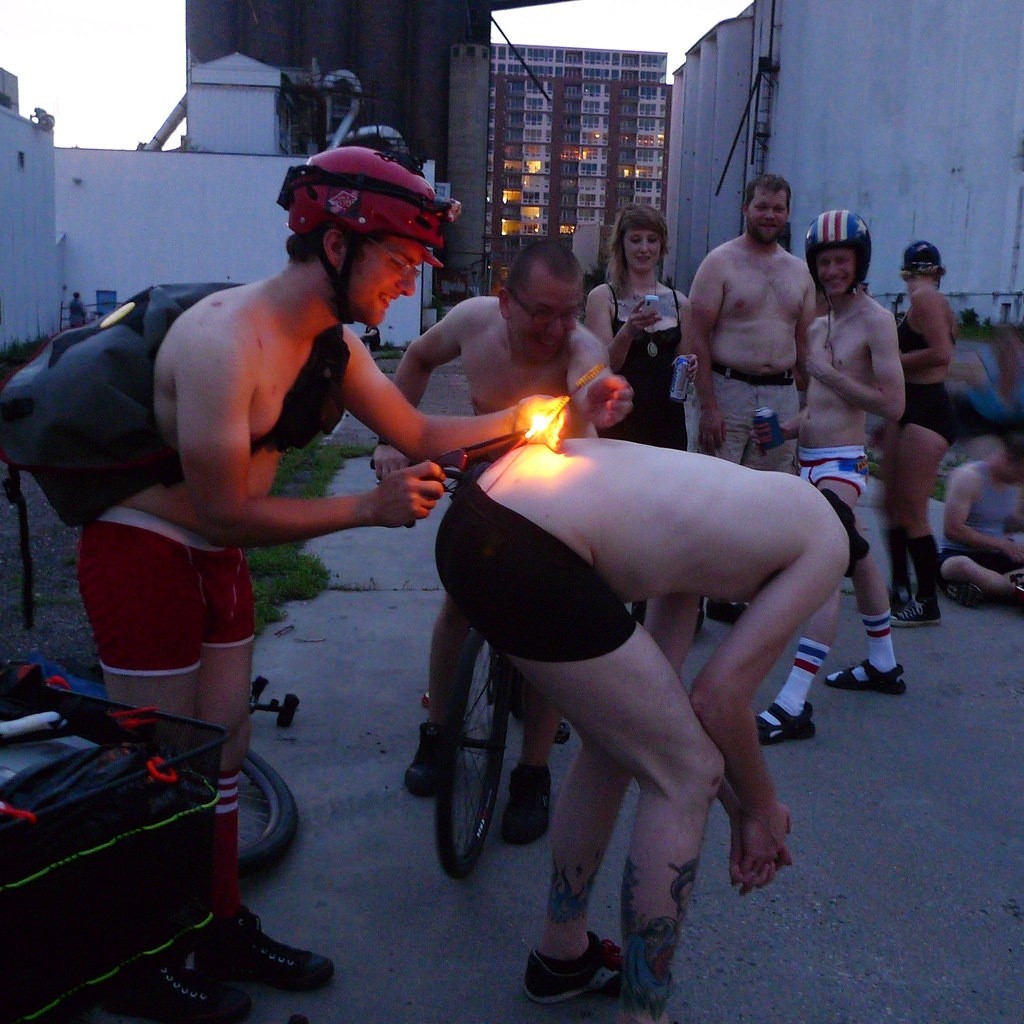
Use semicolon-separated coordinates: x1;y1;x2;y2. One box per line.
1011;572;1024;605
946;580;984;609
706;598;749;623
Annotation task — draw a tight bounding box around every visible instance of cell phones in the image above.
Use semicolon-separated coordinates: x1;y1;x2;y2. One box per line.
643;295;659;315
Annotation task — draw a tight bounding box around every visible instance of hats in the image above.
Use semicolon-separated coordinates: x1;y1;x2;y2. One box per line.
903;240;942;270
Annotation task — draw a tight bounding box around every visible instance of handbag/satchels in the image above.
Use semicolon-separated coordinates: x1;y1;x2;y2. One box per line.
1;281;350;526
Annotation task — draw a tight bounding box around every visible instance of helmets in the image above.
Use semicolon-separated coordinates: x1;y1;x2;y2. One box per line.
291;143;446;269
805;209;871;291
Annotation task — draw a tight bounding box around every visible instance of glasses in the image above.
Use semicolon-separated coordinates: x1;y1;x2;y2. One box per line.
505;283;580;329
361;233;422;286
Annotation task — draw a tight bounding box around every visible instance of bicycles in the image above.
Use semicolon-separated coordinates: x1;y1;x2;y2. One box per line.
367;459;576;881
236;672;302;880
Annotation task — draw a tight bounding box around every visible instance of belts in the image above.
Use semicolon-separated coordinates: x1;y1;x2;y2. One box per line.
709;363;794;385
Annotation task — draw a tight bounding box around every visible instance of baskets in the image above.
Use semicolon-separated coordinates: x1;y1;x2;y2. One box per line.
0;682;225;1023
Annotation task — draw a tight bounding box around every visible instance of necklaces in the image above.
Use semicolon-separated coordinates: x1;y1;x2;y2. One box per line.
632;279;658;356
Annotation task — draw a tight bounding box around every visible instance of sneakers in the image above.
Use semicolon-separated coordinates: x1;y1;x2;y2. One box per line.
889;599;940;628
886;580;913;609
405;720;468;796
525;930;624;1005
106;946;252;1023
503;757;550;842
215;905;333;989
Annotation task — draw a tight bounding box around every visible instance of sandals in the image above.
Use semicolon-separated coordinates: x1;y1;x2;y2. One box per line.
825;659;906;694
754;701;815;745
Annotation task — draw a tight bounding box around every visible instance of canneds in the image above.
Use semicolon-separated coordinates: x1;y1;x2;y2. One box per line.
669;357;692;402
752;407;784;451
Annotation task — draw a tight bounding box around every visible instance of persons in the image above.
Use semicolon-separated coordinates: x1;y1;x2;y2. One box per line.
437;438;852;1024
374;237;634;846
879;241;1023;629
584;203;699;451
74;147;569;1024
689;174;816;624
753;211;906;748
